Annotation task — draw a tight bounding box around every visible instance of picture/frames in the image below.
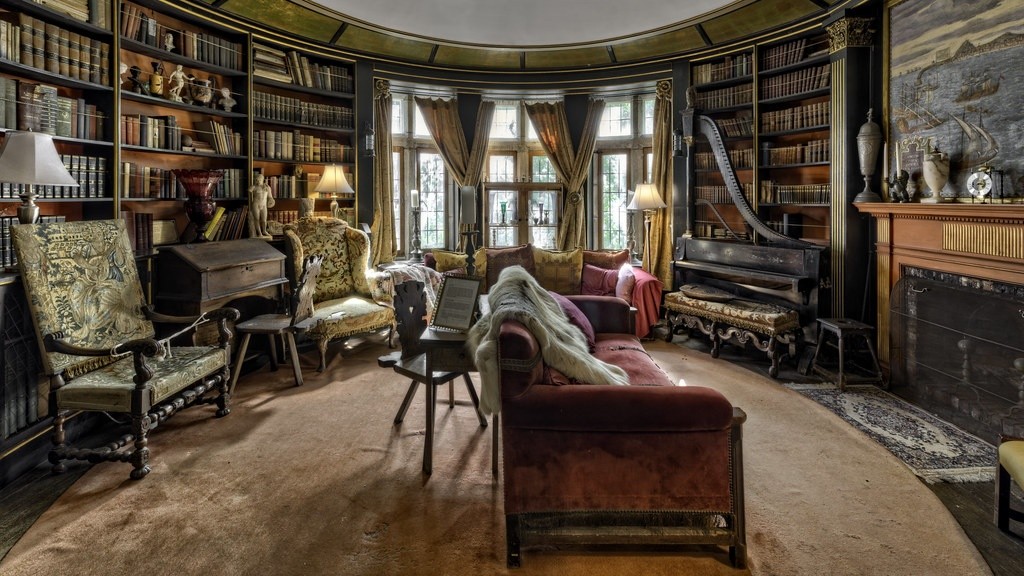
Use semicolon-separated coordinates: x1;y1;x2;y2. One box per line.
881;0;1024;204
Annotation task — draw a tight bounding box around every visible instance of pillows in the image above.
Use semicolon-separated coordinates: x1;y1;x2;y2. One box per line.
427;243;633;303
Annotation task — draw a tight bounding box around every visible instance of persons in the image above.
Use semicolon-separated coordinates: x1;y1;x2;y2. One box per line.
169;64;195;95
218;87;236;108
248;174;273;237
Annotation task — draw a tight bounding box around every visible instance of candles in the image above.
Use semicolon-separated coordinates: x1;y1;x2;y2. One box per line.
884;140;901;179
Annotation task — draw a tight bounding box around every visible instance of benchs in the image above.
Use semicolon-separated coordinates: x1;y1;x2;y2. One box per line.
11;218;240;478
664;292;802;378
473;265;748;571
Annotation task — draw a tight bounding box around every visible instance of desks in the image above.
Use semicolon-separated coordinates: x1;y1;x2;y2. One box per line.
419;325;499;479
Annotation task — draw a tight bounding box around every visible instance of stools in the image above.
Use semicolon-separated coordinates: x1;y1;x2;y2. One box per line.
810;318;885;390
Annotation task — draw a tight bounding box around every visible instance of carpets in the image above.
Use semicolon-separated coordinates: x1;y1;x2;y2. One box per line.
0;338;996;576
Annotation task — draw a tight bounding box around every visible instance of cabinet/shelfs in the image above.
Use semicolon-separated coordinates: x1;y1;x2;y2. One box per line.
0;0;356;445
688;28;832;246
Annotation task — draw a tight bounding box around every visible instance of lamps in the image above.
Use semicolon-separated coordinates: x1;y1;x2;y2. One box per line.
672;128;692;158
458;184;479;274
410;189;424;262
625;184;667;272
316;163;355;217
362;125;376;158
173;168;226;243
0;130;82;272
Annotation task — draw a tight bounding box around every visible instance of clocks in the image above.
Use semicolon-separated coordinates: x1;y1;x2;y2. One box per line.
967;163;1004;204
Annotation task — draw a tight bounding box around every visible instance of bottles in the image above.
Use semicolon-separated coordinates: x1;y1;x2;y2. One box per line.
149;62;164;97
190;79;212;103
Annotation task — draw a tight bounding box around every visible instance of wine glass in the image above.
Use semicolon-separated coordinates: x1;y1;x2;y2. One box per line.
534;218;538;225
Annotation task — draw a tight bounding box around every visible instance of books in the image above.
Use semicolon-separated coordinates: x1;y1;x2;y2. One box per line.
692;38;831;204
0;0;353;441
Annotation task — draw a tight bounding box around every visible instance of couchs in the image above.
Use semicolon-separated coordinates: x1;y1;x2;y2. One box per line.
421;260;662;344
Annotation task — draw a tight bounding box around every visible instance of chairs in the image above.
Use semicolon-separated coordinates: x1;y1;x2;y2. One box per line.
378;279;489;428
283;217;397;373
228;255;325;396
